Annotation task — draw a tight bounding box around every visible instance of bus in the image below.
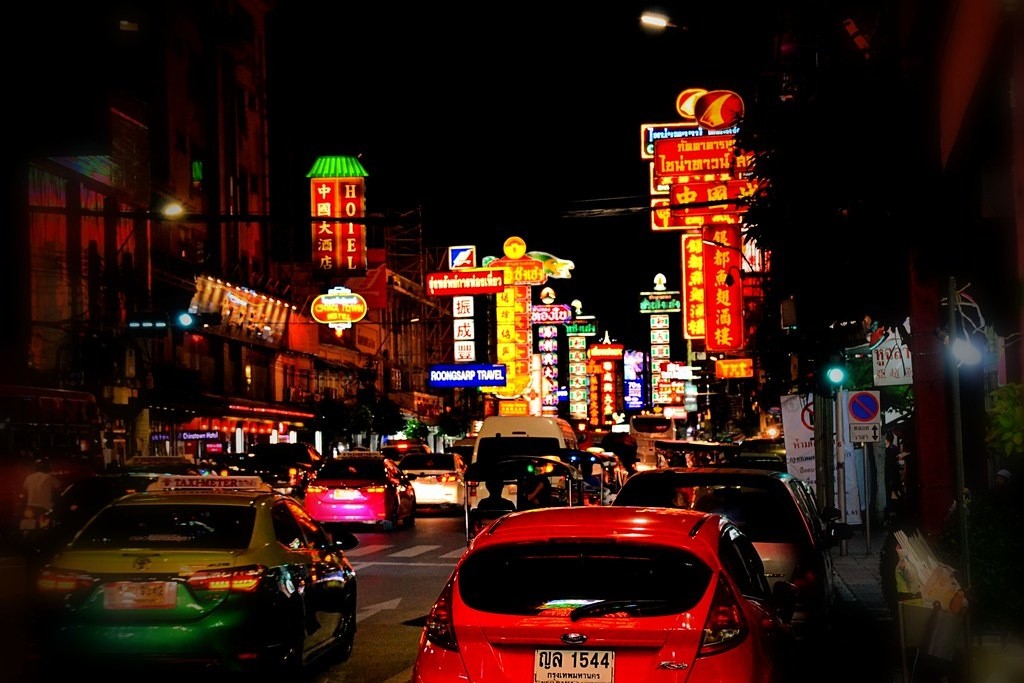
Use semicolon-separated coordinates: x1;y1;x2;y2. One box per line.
628;415;680;473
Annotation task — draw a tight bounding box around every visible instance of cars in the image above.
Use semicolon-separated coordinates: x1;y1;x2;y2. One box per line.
302;451;418;532
464;439;854;640
34;474;357;682
249;443;319;494
36;454;198;552
407;505;797;683
380;437;477;512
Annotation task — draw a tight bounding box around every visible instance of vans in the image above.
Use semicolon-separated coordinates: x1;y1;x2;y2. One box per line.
468;416;580;512
